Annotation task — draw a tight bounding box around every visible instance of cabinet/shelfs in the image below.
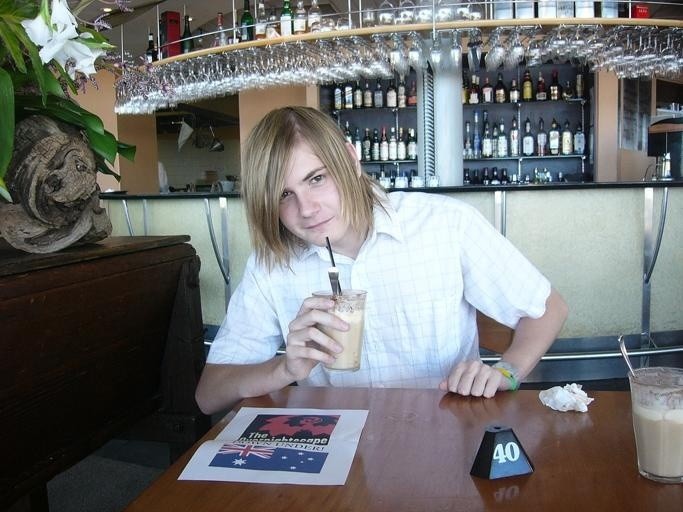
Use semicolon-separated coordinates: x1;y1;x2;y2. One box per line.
319;59;597;191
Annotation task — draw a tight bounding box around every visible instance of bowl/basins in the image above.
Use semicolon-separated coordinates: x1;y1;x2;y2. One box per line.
226;175;237;181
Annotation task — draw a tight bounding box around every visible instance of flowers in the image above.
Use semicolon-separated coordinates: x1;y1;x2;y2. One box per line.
0;0;176;203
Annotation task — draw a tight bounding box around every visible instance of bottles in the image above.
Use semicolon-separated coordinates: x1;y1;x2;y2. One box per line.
144;0;322;63
462;71;584;185
333;79;416;186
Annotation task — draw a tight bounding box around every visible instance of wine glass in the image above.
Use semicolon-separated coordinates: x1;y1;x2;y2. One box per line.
112;0;683;116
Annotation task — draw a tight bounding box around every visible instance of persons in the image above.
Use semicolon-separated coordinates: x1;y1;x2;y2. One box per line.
194;106;569;416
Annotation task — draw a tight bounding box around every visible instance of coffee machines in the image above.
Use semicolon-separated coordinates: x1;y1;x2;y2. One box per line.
646;116;683;182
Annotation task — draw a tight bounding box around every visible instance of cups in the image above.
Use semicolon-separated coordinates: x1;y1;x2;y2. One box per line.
379;176;439;189
312;289;368;372
627;367;683;485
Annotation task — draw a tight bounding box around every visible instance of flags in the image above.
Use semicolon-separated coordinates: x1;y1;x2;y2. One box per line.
210;444;329;474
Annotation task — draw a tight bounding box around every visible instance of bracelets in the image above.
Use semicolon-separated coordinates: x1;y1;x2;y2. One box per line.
490;359;520;394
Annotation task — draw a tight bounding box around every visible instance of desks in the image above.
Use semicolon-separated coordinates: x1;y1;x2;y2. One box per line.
0;233;206;512
